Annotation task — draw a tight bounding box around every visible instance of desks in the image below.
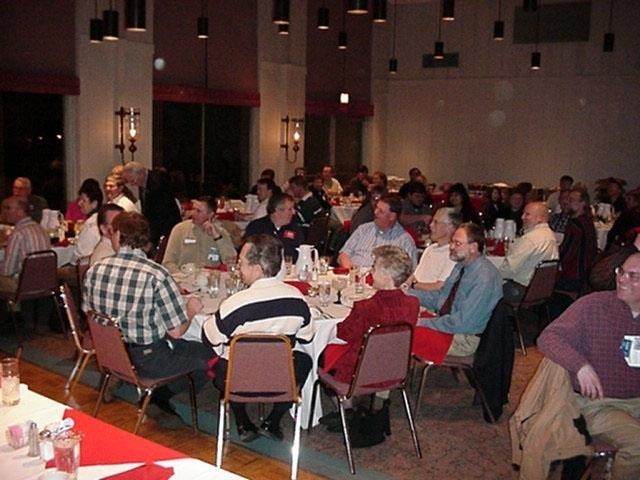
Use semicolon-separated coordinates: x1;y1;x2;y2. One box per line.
2;380;244;480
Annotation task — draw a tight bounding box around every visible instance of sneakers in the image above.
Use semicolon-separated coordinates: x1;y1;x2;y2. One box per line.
144;397;184;431
257;421;283;442
238;423;257;441
102;376;121;402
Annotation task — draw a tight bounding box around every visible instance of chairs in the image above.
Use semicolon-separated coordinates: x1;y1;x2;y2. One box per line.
307;323;422;475
542;356;618;477
4;180;640;368
217;332;302;478
61;284;99;405
409;301;508;437
87;310;198;441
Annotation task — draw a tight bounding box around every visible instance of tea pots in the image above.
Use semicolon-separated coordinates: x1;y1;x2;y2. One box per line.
495;218;505;239
505;220;515;240
46;210;64;228
245;194;257;213
295;244;319;280
597;202;614;220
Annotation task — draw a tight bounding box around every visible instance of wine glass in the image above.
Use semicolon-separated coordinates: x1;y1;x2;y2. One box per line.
332;277;347;304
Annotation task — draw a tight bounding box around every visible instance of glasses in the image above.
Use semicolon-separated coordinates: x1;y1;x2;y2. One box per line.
614;267;640;281
446;240;474;247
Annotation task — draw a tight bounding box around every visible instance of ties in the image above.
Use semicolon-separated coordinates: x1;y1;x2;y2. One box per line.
439;267;463;314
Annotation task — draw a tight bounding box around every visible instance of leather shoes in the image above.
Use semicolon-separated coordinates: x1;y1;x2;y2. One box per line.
319;408;355;423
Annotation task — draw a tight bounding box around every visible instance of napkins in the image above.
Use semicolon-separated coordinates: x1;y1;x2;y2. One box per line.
46;410;189;467
102;460;175;480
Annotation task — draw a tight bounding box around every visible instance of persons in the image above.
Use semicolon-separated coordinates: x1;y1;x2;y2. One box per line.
2;160;640;342
79;211;212;432
88;202;129;404
318;244;422;437
401;219;504;359
534;252;640;478
199;232;315;443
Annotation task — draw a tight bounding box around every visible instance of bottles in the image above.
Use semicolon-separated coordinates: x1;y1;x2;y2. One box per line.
28;422;40;457
40;418;74;439
37;426;55;464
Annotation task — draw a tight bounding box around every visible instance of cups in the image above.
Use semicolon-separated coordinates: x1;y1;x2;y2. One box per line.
54;437;81;480
284;255;292;275
209;271;220;298
352;271;365;293
319;284;330;307
181;262;196;273
0;358;21;407
225;279;235;297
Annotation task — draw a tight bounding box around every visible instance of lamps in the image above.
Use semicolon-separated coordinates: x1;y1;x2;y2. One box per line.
494;0;504;42
271;0;385;34
102;2;118;40
435;4;444;59
339;3;346;52
113;105;143;166
125;2;145;34
199;0;207;39
443;0;456;22
340;93;349;103
602;0;615;49
532;0;541;70
279;113;305;165
89;2;102;43
390;3;397;73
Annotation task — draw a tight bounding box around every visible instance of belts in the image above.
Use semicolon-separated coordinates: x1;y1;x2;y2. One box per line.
126;342;144;347
